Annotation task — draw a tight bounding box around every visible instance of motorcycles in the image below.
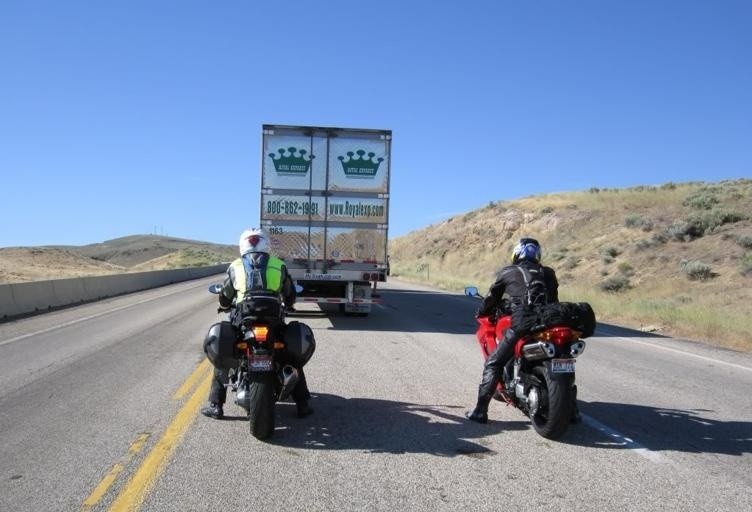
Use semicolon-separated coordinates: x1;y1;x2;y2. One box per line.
204;278;315;439
464;285;586;439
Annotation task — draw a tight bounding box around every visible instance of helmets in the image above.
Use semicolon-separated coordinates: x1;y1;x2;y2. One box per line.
512;238;541;263
239;226;270;257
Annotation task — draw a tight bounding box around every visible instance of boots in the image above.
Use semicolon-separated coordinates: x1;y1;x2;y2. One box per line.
571;385;582;424
297;400;314;419
201;402;223;419
465;384;494;424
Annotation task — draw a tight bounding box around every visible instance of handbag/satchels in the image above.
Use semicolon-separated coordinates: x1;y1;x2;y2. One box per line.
510;302;596;338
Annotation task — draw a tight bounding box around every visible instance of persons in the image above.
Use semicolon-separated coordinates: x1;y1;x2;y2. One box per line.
201;228;315;420
466;238;578;424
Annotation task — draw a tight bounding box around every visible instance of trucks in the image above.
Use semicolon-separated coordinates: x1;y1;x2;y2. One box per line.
260;123;392;316
